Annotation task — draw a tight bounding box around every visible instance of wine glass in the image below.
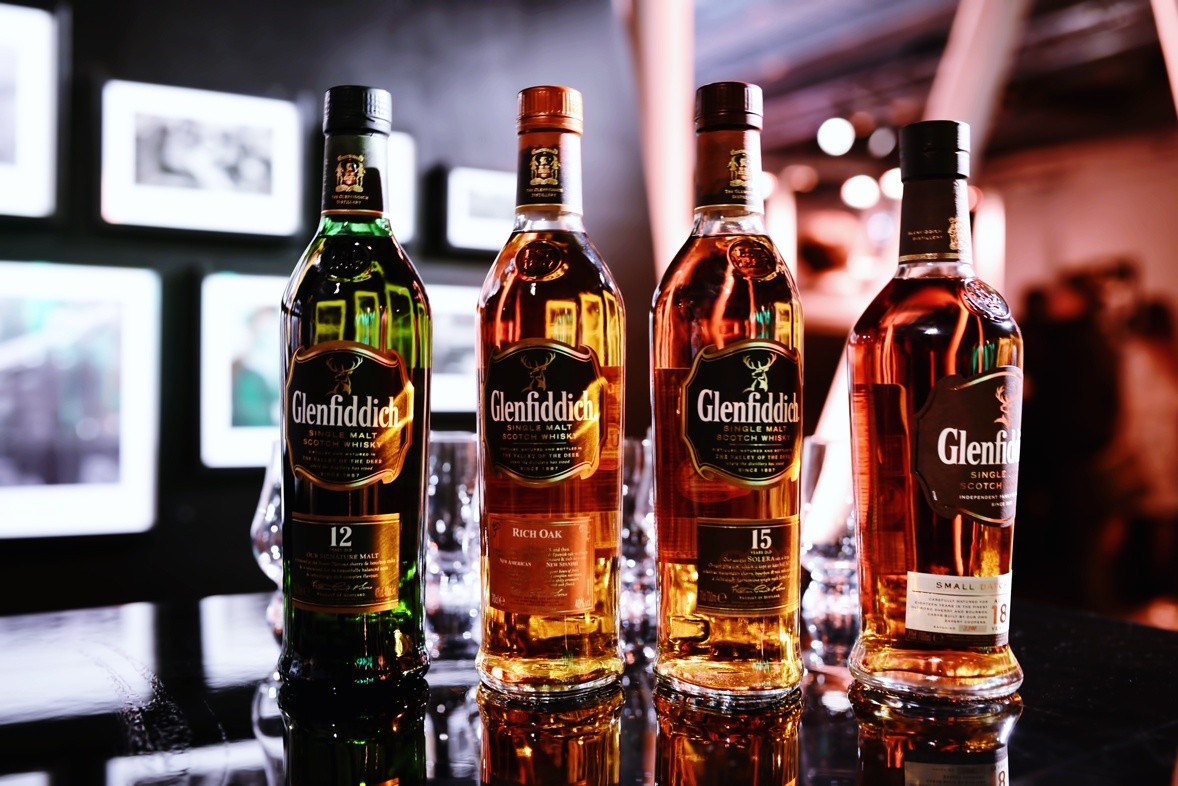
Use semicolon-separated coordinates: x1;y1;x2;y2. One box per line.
423;577;481;667
424;430;481;576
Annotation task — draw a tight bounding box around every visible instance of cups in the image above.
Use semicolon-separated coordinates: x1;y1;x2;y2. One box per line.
799;487;857;585
251;669;286;786
251;429;283;588
619;439;658;653
802;581;861;655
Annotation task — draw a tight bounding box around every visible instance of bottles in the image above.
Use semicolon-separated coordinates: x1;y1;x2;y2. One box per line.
477;681;625;786
847;119;1024;700
272;85;434;694
475;86;627;710
277;694;427;786
647;82;806;701
647;670;804;786
847;680;1024;786
